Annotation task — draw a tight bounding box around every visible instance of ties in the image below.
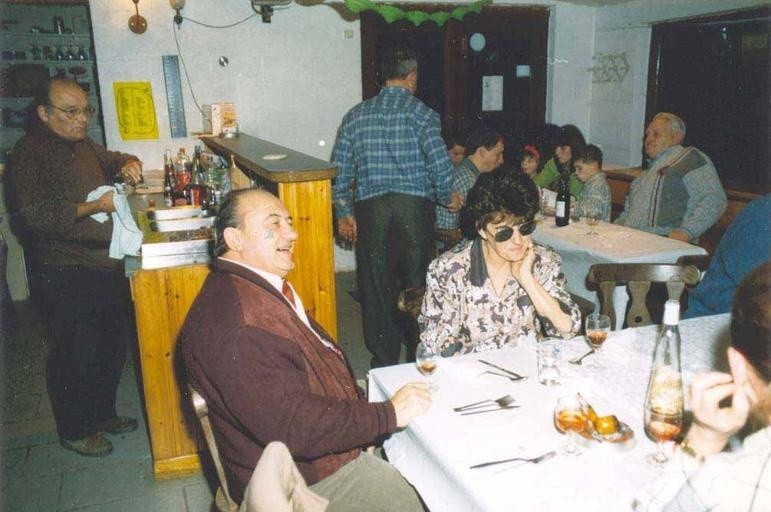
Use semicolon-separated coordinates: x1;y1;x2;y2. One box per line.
285;280;298;309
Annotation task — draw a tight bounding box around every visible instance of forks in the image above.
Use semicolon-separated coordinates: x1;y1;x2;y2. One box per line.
471;452;558;470
454;396;519;412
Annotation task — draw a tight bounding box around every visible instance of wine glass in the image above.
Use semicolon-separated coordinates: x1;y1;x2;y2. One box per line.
643;406;683;467
586;312;611;372
539;196;548;220
586;210;599;236
416;345;440;391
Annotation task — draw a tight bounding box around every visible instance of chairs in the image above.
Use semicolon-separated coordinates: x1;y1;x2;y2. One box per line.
587;263;701;331
186;380;239;512
435;228;461;257
397;287;427;364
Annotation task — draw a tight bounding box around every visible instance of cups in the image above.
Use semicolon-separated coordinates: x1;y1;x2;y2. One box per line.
537;339;563;386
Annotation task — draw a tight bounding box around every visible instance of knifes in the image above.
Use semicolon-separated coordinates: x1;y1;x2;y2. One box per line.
481;359;523;378
460;406;520;416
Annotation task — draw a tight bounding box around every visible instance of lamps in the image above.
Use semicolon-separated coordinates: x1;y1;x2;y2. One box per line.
128;0;147;35
168;0;186;27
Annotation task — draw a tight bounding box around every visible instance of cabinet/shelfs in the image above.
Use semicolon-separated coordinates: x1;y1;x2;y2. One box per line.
124;132;338;482
0;0;107;173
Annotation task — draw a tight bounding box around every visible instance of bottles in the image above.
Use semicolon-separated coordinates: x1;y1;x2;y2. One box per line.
555;170;570;226
164;149;177;200
165;173;175;208
643;299;684;444
175;146;192;197
189;144;232;211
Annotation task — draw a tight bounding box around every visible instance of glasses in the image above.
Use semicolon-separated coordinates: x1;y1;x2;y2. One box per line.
50;102;98;117
483;221;538;242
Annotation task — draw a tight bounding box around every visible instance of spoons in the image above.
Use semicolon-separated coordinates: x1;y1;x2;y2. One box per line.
568;350;598;366
479;372;529;382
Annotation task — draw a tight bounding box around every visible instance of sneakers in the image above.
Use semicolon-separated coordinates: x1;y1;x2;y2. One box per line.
63;431;114;456
101;415;140;435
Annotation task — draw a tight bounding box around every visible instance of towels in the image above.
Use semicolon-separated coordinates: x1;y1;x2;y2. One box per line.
86;183;143;260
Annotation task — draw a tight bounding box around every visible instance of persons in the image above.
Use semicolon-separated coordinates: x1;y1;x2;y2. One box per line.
434;131;505;256
182;189;438;510
612;113;727;329
418;167;582;359
12;78;144;456
679;189;770;319
570;143;611;223
329;49;464;368
441;132;465;166
520;143;550;178
532;124;587;195
631;260;771;512
